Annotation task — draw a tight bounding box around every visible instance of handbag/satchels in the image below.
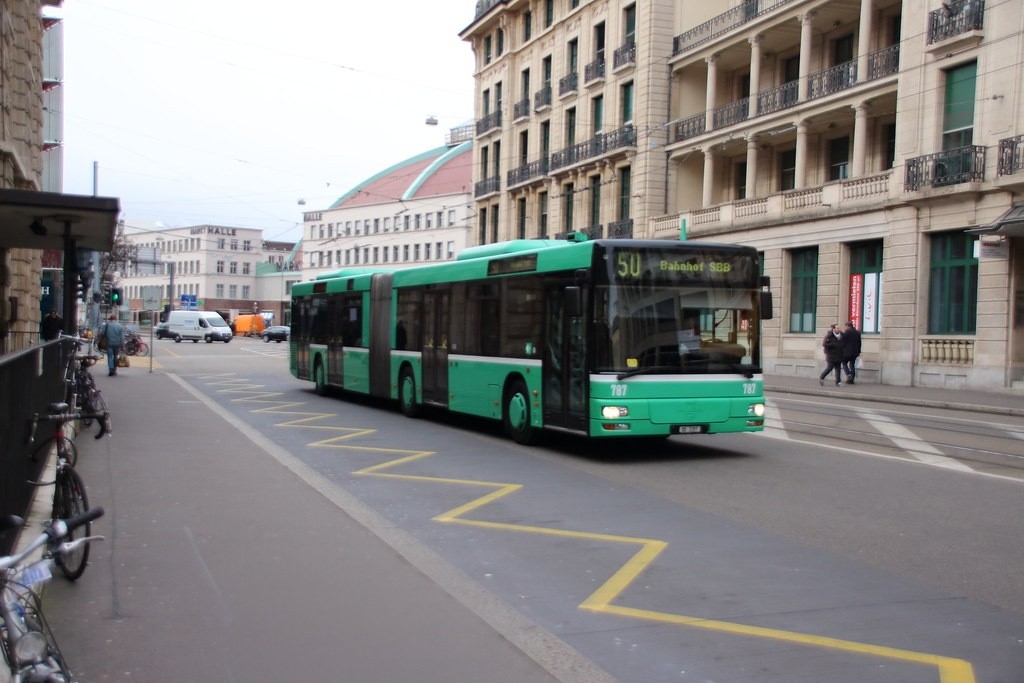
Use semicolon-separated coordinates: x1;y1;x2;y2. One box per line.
118;351;130;367
854;355;863;368
98;323;109;350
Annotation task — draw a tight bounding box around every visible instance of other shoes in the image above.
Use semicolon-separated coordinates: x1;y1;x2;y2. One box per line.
845;377;854;384
108;369;117;376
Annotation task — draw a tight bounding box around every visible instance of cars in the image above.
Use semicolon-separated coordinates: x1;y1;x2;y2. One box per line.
261;326;290;343
155;324;178;340
153;322;162;333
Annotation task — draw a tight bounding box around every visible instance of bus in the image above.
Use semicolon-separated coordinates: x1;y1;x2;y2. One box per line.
289;238;773;444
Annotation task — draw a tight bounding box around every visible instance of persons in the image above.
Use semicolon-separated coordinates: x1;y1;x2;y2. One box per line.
819;321;862;387
41;310;63;342
680;316;702;345
101;315;125;376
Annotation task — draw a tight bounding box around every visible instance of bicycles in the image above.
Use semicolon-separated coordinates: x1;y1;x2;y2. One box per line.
59;332;113;433
93;327;149;357
27;401;109;580
0;505;105;683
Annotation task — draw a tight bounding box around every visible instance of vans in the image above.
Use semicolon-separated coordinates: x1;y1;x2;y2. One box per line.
226;315;265;337
168;310;233;343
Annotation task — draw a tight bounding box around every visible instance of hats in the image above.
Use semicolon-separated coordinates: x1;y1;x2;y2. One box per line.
110;315;117;320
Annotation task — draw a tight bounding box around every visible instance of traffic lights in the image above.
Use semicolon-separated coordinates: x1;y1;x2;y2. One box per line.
111;288;123;307
